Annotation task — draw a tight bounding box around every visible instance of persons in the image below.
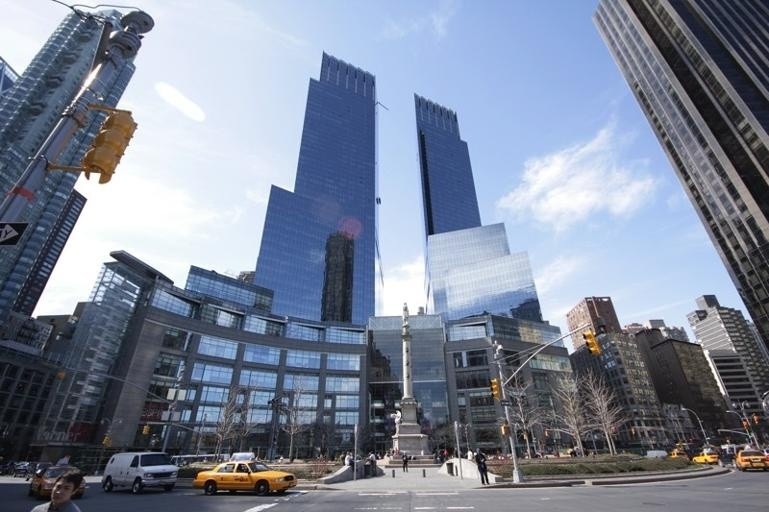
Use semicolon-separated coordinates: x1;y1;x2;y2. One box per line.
432;448;449;464
401;451;408;472
474;448;490;485
716;448;735;467
467;448;473;461
30;471;82;512
340;447;394;477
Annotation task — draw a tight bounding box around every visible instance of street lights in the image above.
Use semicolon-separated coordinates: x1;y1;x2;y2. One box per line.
681;406;709;446
725;410;753;448
492;340;525;482
458;423;473;451
733;397;760;450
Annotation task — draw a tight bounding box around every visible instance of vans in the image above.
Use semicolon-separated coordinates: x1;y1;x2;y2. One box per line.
718;445;747;467
101;451;179;494
26;461;54;481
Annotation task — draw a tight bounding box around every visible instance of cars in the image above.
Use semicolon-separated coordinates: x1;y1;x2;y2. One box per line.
29;456;86;499
668;450;691;464
736;450;769;471
760;448;769;454
693;451;719;465
192;451;297;495
0;459;15;477
14;461;29;476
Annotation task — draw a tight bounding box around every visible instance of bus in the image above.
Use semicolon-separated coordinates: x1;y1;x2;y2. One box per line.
170;452;230;466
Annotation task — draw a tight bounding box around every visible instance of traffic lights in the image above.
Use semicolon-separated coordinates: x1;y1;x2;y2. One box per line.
502;426;510;435
143;426;146;434
489;377;501;401
582;327;601;356
146;426;150;435
